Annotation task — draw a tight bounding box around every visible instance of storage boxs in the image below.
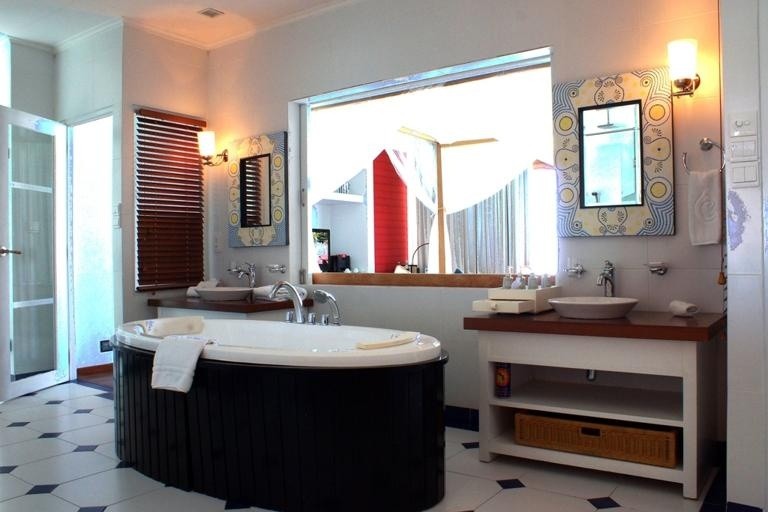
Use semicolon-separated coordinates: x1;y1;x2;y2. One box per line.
515;410;678;470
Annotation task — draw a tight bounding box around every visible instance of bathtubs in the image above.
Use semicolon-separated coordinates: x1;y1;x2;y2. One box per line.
112;318;443;508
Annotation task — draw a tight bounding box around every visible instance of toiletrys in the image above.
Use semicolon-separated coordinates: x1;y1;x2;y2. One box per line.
501;265;551;291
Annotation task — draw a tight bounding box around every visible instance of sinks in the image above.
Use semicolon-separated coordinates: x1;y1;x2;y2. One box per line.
193;283;253;302
545;294;640;318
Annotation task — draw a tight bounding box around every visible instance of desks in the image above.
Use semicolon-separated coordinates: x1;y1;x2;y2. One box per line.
464;310;726;500
148;289;314;323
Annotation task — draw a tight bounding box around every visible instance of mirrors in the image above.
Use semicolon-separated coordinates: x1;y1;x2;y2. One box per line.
300;54;558;287
552;66;675;238
226;130;288;249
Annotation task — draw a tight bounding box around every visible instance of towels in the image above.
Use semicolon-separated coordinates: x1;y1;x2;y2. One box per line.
254;284;308;303
668;299;699;317
687;169;722;246
150;333;218;396
186;278;221;298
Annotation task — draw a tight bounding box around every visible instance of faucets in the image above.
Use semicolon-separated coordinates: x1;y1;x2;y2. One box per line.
410;241;430;266
268;280;306;322
595;258;617;297
227;259;256;302
312;289;341;323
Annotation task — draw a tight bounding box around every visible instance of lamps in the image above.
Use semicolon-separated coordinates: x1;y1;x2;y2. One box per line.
196;130;229;167
666;37;701;98
